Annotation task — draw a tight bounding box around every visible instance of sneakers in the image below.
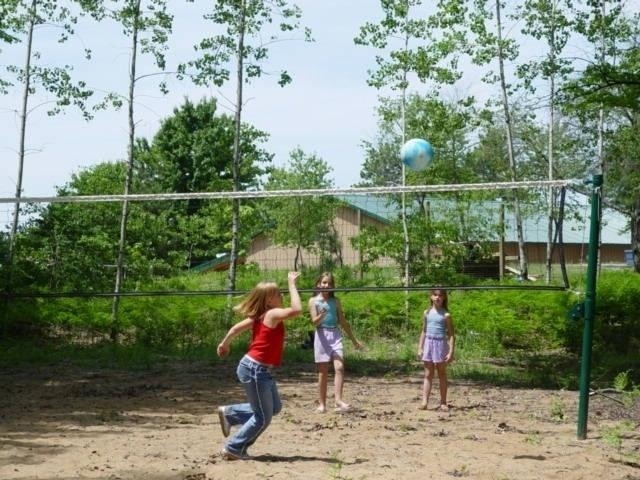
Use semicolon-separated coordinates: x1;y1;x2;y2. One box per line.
217;405;232;438
221;447;254;461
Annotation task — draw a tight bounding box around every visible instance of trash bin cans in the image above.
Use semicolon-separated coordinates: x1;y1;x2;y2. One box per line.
624;250;634;266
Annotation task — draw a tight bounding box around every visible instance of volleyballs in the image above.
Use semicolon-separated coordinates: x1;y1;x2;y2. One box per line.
401;138;433;170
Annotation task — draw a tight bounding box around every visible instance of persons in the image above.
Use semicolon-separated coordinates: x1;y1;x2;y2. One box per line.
216;271;303;460
417;284;454;410
308;271;364;413
